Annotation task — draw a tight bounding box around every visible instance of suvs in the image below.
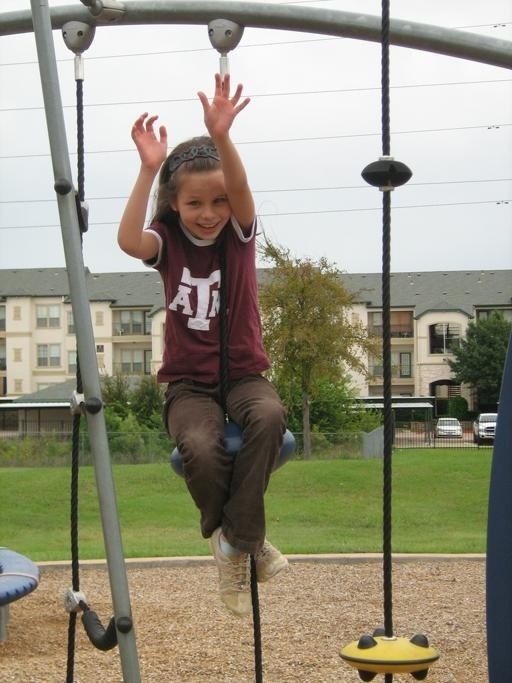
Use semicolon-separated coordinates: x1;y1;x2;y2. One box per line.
472;412;498;443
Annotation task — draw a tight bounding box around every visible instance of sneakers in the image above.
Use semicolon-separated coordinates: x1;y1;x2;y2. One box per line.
211;526;287;616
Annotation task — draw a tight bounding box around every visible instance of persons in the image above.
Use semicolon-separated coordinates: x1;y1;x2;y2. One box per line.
117;73;290;617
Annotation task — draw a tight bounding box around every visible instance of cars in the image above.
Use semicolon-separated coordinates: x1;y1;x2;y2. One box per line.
433;416;463;437
